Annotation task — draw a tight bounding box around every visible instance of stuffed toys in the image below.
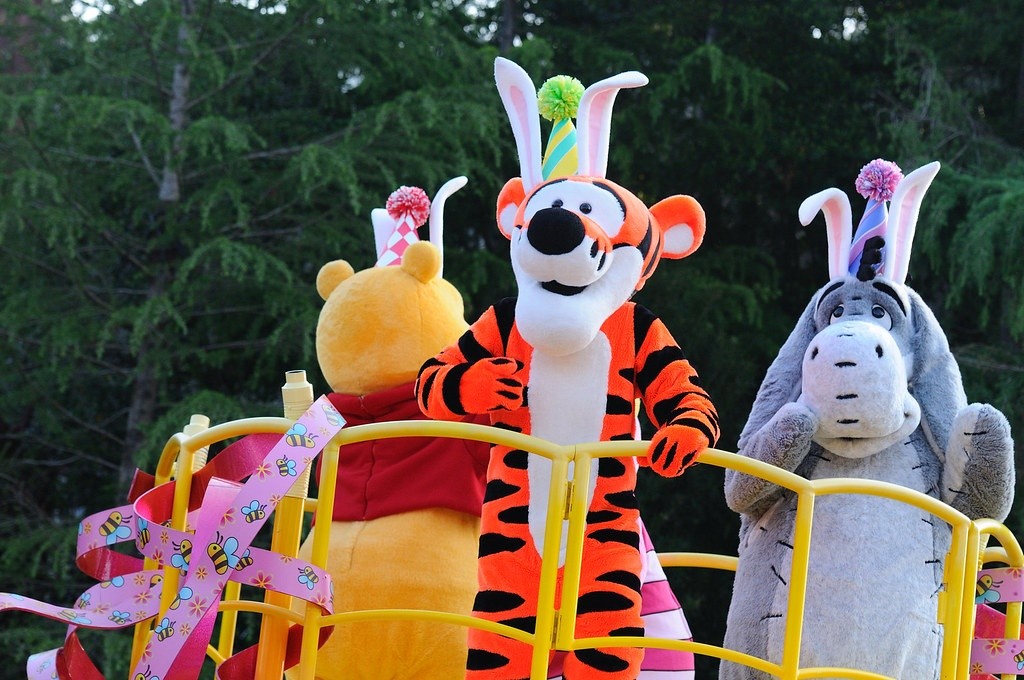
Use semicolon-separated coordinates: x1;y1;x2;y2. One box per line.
632;415;698;680
414;58;721;680
214;175;487;680
718;156;1016;680
